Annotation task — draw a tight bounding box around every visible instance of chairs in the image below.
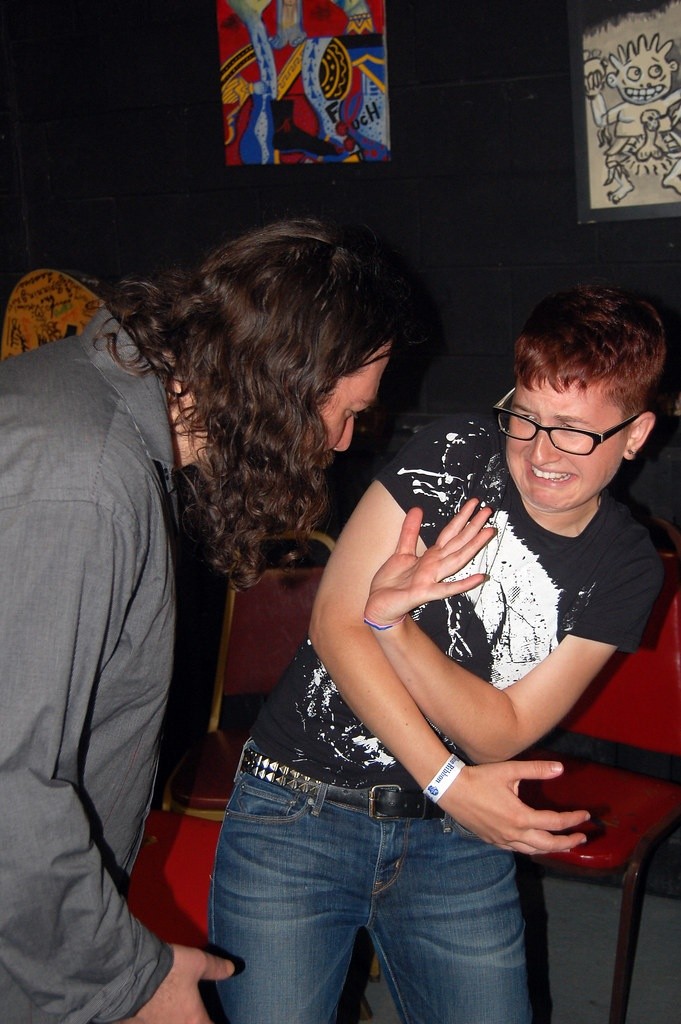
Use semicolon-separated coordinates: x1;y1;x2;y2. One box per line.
514;514;680;1024
162;526;383;987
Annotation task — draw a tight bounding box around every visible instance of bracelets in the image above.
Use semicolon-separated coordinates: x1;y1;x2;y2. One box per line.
423;753;465;804
364;614;407;630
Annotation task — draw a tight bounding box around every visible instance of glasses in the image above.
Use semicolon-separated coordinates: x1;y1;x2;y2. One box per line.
492;386;652;455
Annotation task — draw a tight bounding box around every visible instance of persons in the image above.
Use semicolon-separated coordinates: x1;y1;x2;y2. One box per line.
205;287;667;1024
0;221;417;1024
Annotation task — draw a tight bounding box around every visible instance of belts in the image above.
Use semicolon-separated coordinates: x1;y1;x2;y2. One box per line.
241;748;446;820
